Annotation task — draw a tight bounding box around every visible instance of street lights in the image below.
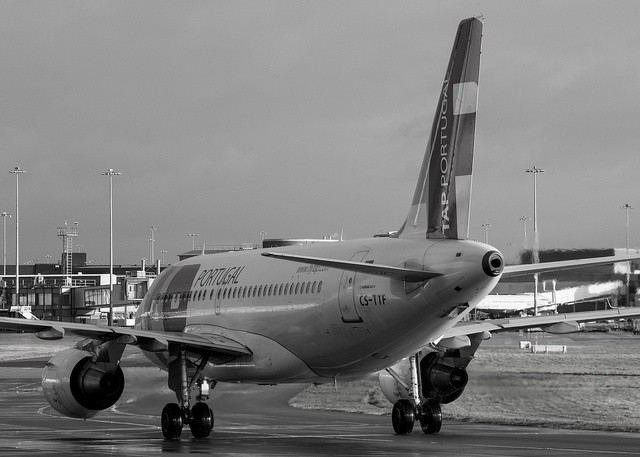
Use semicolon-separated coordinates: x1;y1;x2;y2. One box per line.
618;204;633;309
148;225;157;263
481;221;491;242
524;165;544;230
102;168;122;289
160;249;167;265
519;215;530;254
188;232;198;249
8;166;26;291
0;211;11;273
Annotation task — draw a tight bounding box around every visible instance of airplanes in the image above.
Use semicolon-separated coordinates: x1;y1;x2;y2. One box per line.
0;16;640;437
